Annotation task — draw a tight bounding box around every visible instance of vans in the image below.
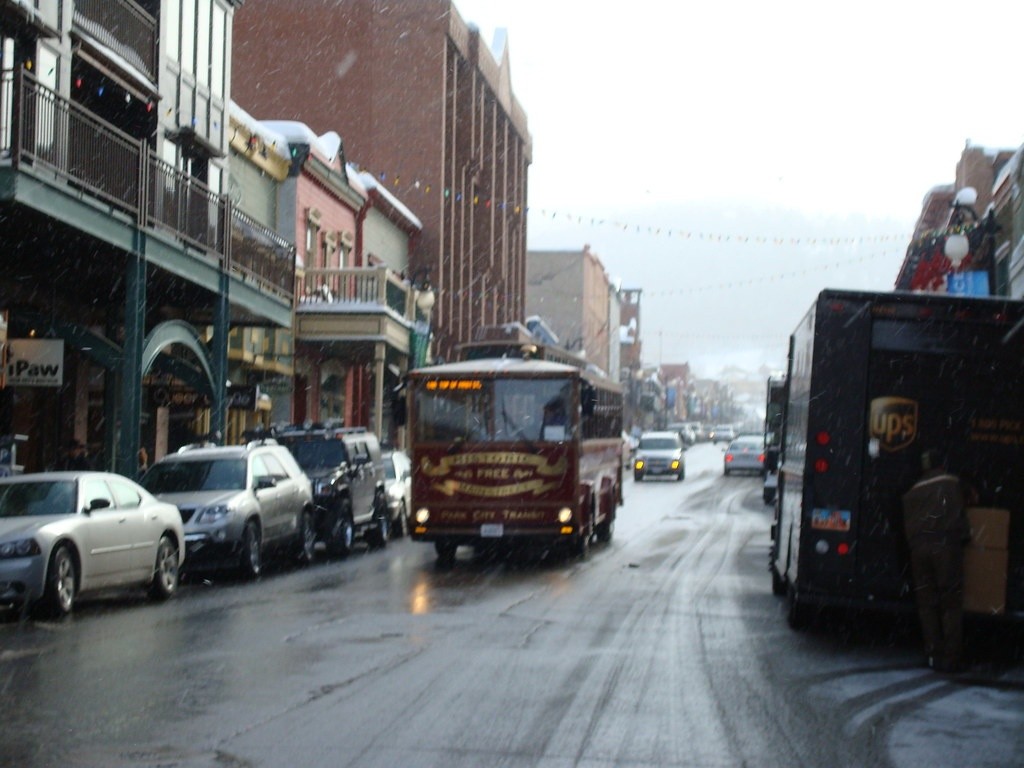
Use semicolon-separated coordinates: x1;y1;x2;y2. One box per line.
629;431;687;482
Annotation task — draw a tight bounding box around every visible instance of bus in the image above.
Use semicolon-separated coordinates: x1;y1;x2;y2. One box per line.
393;345;626;563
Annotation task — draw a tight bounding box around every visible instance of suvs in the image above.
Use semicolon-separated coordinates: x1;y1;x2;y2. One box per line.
138;437;318;578
241;416;390;557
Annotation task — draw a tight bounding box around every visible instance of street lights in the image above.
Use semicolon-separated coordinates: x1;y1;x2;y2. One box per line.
944;187;999;298
401;265;436;364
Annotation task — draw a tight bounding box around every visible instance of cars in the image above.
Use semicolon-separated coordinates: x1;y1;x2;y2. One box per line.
0;470;186;615
665;422;696;449
704;424;715;442
720;436;765;475
734;431;763;439
380;451;412;537
690;422;706;442
712;425;735;444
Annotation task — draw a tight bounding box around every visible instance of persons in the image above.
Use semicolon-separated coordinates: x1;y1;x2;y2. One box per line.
904;448;968;671
25;439;148;479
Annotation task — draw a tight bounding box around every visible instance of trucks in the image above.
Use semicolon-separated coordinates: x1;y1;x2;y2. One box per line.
769;288;1024;645
763;375;785;504
